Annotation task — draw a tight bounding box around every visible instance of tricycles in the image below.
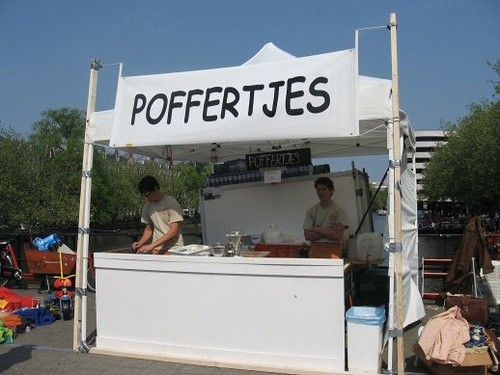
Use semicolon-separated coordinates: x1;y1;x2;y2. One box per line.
48;274;76;321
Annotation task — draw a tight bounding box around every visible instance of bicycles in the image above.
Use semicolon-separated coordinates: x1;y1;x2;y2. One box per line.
0;238;96;293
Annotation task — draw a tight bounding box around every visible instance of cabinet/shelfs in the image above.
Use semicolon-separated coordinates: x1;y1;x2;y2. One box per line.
256;243;307;259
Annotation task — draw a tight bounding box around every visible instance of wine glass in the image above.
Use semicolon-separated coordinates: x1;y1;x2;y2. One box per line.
229;230;241;257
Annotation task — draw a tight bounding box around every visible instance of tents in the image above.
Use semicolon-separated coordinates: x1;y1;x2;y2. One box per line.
72;11;426;375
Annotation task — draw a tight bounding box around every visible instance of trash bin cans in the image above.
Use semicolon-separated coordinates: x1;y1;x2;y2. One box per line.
346;306;385;373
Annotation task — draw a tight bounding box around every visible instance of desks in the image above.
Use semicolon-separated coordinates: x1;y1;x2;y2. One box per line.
413;326;500;375
94;248;351;369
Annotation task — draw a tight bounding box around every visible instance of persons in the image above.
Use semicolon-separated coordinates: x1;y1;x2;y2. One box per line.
303;176;350;259
132;175;185;255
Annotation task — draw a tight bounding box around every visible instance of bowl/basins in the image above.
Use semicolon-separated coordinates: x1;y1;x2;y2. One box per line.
209;244;236;256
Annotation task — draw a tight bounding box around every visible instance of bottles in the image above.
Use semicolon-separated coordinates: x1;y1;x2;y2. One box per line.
60;287;72;321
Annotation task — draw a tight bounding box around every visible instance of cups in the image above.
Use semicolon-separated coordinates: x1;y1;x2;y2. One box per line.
32;297;46;309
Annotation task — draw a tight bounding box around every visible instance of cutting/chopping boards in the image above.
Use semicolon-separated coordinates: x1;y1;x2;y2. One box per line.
168;244;213;255
